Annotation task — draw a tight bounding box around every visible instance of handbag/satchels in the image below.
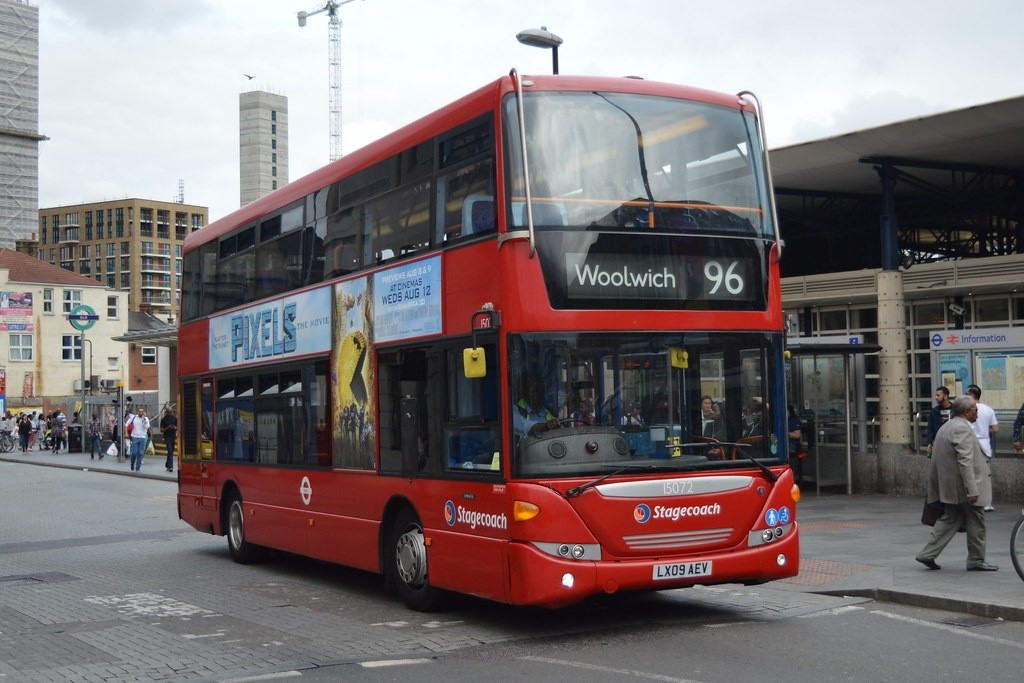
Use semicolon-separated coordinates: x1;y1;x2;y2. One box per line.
921;496;969;533
145;440;155;456
107;442;118;458
126;423;133;435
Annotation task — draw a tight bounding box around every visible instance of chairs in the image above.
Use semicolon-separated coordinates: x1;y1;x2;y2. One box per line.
522;203;564;227
462;191;496;235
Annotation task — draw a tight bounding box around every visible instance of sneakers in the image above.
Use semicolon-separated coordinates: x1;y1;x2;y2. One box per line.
984;505;994;512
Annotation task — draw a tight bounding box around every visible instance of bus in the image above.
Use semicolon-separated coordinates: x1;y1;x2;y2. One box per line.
175;74;802;615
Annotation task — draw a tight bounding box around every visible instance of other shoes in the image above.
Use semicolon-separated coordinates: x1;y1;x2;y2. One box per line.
165;463;168;471
130;463;134;471
168;467;173;472
99;455;104;460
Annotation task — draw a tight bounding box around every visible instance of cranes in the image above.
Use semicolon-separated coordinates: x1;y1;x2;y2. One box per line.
296;0;355;163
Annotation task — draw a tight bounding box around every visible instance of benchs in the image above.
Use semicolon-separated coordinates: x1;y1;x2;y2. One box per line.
788;450;807;489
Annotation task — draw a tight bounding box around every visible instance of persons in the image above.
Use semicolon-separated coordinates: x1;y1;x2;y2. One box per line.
112;410;152;458
86;412;104;460
740;401;801;483
915;395;999;571
72;411;82;424
0;408;67;454
1013;402;1024;450
571;394;644;428
513;383;560;437
342;399;365;451
160;408;177;472
965;384;999;512
124;409;150;472
927;386;956;459
18;414;32;453
701;395;727;460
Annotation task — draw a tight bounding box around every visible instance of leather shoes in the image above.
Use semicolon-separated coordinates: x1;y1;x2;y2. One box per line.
915;555;941;570
967;560;1000;572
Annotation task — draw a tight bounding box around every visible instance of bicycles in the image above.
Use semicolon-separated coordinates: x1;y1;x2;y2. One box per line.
1009;448;1024;583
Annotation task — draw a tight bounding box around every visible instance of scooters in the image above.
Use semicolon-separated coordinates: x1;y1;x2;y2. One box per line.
0;424;16;453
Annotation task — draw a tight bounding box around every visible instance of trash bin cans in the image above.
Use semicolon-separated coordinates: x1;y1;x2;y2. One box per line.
68;424;82;453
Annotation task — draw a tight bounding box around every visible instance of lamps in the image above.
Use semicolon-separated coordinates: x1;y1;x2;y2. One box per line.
897;248;914;268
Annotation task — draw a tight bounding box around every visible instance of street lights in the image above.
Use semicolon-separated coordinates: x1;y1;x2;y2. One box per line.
77;337;93;396
